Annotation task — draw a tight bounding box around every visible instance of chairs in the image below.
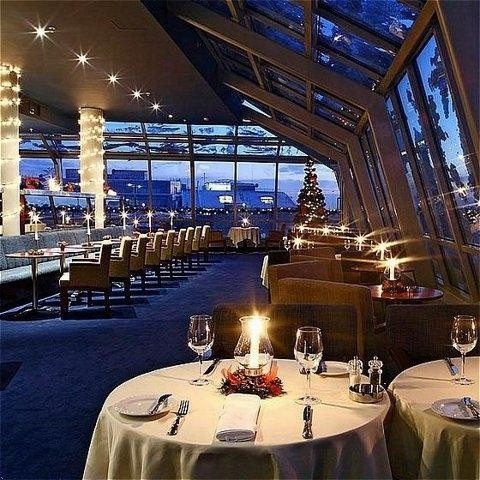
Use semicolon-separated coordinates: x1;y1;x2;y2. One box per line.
207;230;227;255
58;225;210;320
260;222;480;384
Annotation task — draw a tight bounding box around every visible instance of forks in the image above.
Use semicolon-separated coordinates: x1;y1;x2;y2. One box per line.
168;400;189;436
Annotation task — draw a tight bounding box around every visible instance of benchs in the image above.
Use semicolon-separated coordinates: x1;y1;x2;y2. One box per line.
0;225;133;314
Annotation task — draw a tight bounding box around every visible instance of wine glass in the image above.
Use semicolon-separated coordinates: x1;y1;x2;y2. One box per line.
293;325;323;406
449;314;478;386
187;313;217;386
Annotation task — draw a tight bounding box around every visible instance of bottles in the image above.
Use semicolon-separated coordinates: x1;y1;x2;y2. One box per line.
347;355;362;385
367;355;383;385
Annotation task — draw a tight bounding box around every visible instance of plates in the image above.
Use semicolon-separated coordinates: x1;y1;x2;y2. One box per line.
429;398;478;421
113;394;179;417
312;360;353;378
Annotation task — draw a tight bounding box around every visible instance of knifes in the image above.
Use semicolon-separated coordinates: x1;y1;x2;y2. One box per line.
150;393;173;416
302;405;313;440
203;356;222;377
443;352;458;377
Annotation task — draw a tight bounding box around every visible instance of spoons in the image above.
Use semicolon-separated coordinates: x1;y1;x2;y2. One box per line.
462;396;477;418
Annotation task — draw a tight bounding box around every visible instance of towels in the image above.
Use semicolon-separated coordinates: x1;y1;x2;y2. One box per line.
215;393;261;442
